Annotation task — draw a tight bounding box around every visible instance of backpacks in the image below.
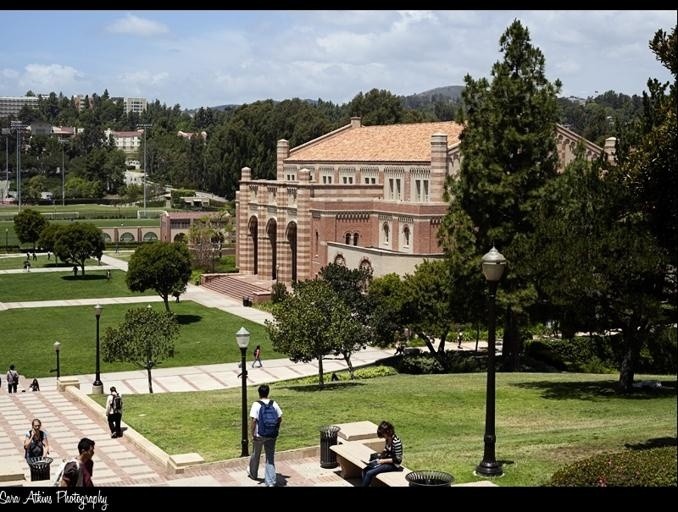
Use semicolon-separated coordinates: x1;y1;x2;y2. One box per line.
255;400;279;438
110;394;122;412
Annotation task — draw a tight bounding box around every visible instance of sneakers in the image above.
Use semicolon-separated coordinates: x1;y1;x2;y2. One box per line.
247;466;257;479
111;432;116;437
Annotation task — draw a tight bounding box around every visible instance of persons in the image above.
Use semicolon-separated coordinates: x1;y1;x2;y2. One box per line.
393;339;406;355
360;421;403;488
106;268;113;281
29;379;40;392
114;242;121;253
22;251;53;272
60;438;95;487
6;366;18;393
252;345;263;368
24;419;49;458
70;265;80;280
247;384;283;487
457;329;464;348
106;386;124;441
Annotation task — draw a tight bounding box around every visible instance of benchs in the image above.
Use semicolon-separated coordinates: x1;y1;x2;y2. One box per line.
329;440;415;487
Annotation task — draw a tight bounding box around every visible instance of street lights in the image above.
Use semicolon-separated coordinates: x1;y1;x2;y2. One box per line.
53;341;62;380
92;304;104;395
136;124;153;217
5;228;9;247
276;265;280;284
11;121;27;211
2;127;10;179
475;245;508;478
57;140;70;206
211;248;216;271
236;325;251;457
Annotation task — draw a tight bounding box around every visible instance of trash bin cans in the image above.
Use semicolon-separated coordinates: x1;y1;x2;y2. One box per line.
195;280;199;285
319;425;341;468
27;457;53;481
404;470;454;487
243;295;249;306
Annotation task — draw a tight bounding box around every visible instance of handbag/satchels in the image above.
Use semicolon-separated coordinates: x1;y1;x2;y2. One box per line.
13;376;18;384
